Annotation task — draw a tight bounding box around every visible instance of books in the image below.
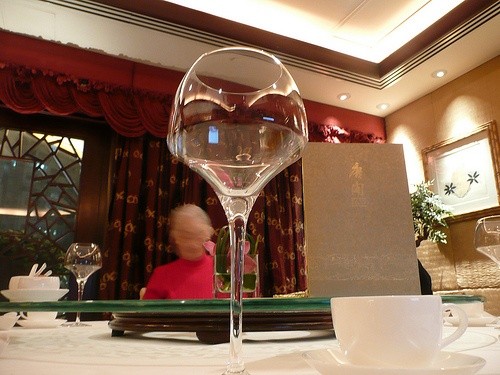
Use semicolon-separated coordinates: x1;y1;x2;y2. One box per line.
300;142;420;296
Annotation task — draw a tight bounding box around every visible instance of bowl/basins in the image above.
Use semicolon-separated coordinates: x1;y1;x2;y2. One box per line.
0;316;19;329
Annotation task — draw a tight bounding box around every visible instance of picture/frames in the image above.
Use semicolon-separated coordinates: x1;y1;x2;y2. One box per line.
421;119;500;226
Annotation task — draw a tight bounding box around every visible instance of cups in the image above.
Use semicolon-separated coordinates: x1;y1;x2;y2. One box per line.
19;311;58;320
330;296;467;365
10;276;60;290
474;216;500;265
446;302;484;317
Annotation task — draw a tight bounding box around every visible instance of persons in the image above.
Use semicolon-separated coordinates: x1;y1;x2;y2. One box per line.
140;205;247;299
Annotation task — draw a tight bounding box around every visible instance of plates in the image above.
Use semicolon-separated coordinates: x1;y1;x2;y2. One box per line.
1;289;69;302
303;349;486;375
18;320;67;328
444;316;496;326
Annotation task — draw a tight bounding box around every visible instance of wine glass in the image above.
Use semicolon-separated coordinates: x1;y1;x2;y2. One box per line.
167;48;308;375
61;243;103;327
